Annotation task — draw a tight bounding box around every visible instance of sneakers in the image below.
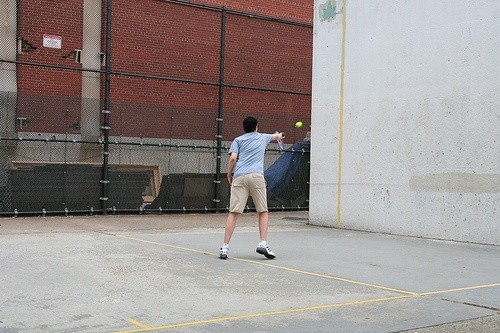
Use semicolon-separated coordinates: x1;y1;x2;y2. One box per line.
219;247;229;259
256;243;275;259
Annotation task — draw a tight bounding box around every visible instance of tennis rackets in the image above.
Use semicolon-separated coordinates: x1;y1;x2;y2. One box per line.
276;131;285;150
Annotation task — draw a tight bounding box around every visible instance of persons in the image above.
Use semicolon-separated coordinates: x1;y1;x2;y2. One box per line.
219;116;285;259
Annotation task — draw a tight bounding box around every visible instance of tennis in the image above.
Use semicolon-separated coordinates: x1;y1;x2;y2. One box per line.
295;121;302;127
